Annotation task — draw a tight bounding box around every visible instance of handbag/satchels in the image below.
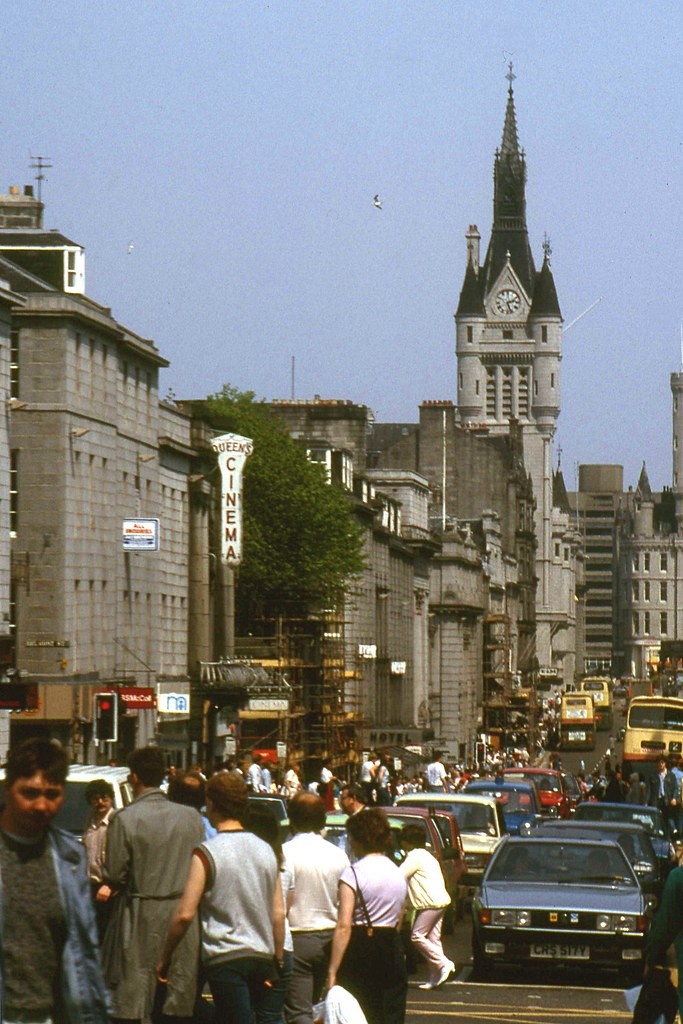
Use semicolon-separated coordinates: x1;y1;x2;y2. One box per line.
374;937;408;981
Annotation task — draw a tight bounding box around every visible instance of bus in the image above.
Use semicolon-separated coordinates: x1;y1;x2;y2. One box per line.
561;691;596;751
628;680;653;700
580;678;614;730
623;695;683;775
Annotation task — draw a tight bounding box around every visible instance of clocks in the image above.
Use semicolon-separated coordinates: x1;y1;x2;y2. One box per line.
494;290;521;315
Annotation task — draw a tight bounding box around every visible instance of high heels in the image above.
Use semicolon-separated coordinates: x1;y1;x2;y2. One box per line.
419;978;437;988
435;960;455;986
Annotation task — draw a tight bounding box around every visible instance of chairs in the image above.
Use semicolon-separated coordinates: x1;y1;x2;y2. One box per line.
581;851;614;878
615;834;637;860
503;847;532;877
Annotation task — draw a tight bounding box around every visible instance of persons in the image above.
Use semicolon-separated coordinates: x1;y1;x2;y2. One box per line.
155;771;284;1024
281;791;350;1024
106;747;207;1024
327;810;408;1024
241;804;295;985
0;739;114;1024
588;852;610;875
645;866;683;1024
511;690;565;770
618;835;637;862
396;751;523;793
428;749;448;793
82;779;115;943
167;752;393;865
577;755;683;844
540;779;551;790
399;824;456;989
472;807;495;835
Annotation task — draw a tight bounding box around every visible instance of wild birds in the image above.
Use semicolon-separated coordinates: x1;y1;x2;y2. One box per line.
371;194;384;210
127;240;136;254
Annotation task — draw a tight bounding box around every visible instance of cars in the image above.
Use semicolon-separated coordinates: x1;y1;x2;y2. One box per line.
464;781;539;839
498;767;581;822
391;794;501;886
459;839;649;981
244;798;469;937
534;800;677;913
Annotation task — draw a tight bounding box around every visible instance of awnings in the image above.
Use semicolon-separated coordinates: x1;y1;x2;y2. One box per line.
376;746;429;766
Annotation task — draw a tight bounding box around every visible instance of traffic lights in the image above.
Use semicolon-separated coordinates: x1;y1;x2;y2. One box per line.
476;742;486;763
93;693;117;742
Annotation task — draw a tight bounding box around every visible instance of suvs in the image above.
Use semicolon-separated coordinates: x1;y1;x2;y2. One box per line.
0;765;132;840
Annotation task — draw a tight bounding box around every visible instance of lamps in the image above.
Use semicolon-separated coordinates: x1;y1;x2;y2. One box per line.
138;454;155;463
69;428;89;438
3;400;28;411
188;475;204;483
